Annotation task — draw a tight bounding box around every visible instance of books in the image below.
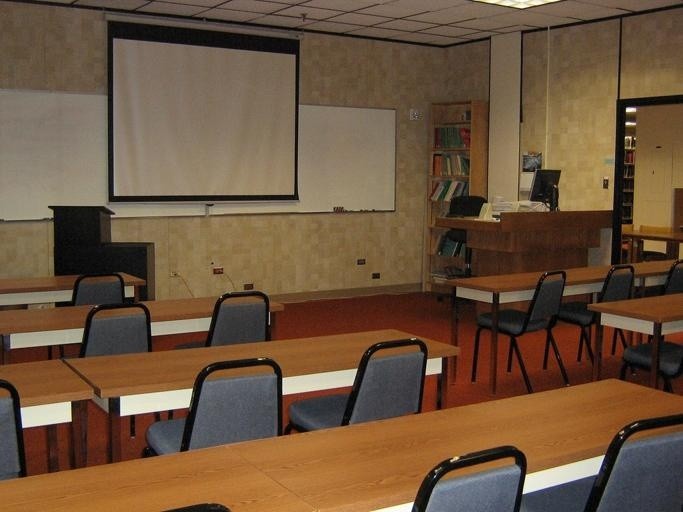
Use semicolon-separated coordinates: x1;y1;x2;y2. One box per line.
621;135;635;223
429;125;470;259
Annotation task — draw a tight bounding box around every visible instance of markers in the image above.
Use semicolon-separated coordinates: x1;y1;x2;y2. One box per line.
360;208;376;211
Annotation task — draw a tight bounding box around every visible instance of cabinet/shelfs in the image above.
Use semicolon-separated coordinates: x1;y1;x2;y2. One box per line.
622;136;634;224
424;99;486;282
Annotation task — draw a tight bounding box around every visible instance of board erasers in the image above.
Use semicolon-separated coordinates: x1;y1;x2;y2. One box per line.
332;206;344;211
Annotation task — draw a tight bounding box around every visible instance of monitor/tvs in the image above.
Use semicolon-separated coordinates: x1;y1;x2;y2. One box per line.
528;169;562;212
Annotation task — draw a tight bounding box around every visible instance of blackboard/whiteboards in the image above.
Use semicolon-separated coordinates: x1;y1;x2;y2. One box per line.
0;89;396;222
